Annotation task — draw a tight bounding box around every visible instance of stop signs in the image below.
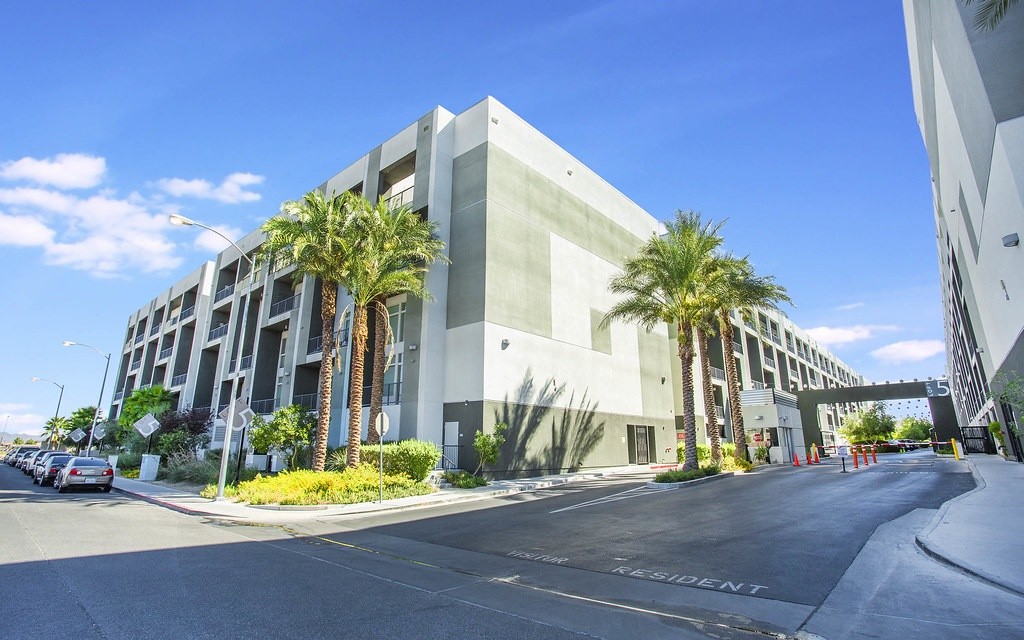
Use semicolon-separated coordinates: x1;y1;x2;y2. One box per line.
753;433;762;441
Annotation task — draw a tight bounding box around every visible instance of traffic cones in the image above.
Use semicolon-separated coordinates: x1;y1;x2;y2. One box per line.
806;452;815;466
792;453;802;467
813;451;821;463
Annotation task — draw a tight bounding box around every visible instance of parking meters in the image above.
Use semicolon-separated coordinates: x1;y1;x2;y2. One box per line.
765;439;773;465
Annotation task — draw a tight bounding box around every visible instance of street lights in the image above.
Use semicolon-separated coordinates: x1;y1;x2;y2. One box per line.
31;377;64;450
63;339;112;457
168;213;257;501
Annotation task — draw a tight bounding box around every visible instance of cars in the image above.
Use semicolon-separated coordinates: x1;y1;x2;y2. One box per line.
32;456;81;487
2;445;73;479
54;457;114;493
877;439;920;451
